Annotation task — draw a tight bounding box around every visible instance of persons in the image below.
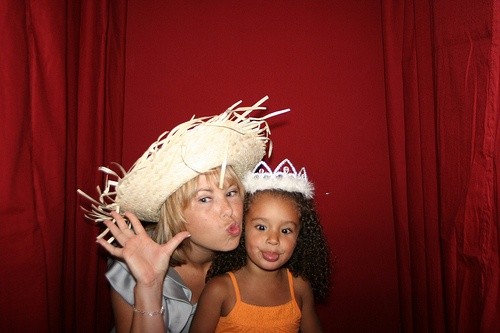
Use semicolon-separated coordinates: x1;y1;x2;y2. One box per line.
187;159;323;333
76;95;291;332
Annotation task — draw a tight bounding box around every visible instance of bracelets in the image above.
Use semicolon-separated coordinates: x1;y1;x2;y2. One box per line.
133;304;165;316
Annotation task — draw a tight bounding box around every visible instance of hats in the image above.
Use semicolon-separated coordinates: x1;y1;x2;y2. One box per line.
241;157;315;200
75;95;291;245
